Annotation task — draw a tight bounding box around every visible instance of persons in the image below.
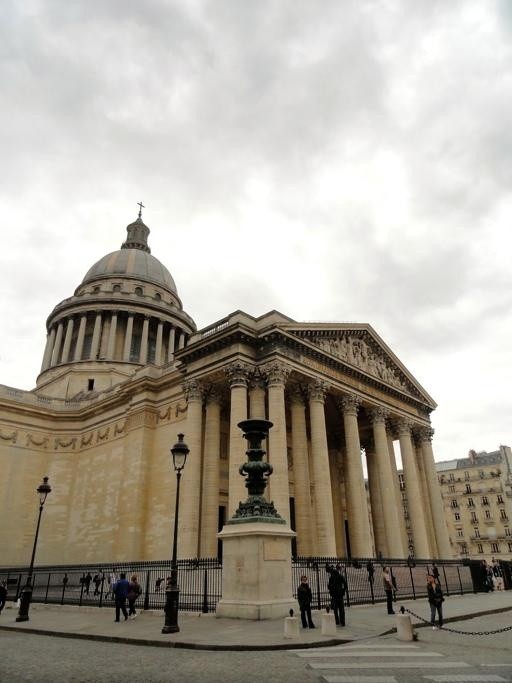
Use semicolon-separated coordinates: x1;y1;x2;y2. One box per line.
382;565;400;615
297;575;317;630
112;574;129;623
481;556;503;592
105;567;118;600
93;569;104;596
126;575;142;620
82;571;91;595
408;544;416;567
427;576;446;630
0;578;8;615
326;569;350;626
17;592;23;611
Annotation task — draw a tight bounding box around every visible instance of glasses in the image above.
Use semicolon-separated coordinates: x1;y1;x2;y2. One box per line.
302;579;307;582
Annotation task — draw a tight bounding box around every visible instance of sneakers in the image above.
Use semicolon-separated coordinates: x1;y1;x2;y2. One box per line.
131;614;137;620
433;625;445;631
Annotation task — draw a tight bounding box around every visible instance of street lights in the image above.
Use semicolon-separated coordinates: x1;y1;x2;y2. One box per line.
15;476;52;621
162;433;190;632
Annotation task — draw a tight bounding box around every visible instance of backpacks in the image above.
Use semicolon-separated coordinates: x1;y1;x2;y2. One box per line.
135;583;142;594
